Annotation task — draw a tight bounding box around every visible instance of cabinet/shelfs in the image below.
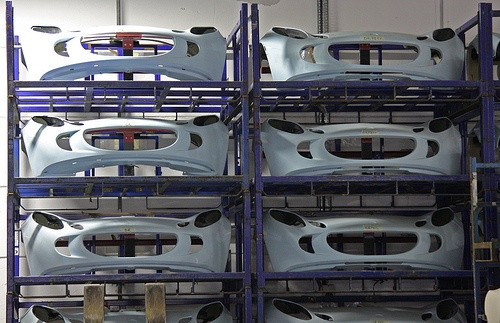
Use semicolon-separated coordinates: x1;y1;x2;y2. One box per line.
6;0;500;323
